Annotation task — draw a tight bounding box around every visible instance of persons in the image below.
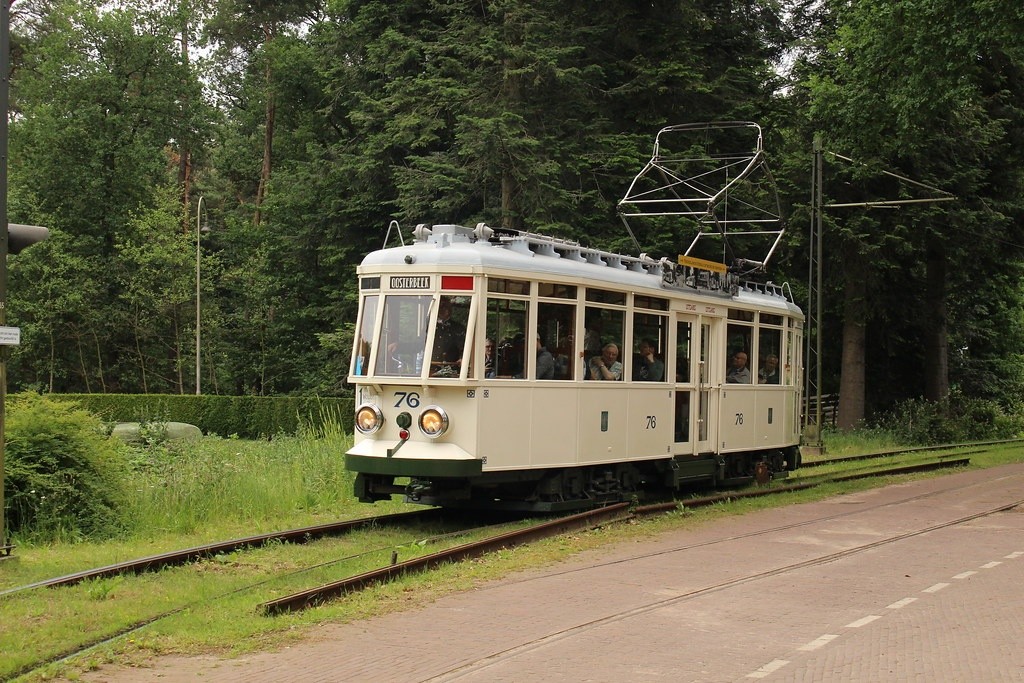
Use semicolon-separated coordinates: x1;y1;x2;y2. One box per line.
759;353;779;384
511;332;554;379
632;338;664;381
387;296;467;374
485;337;503;378
726;352;750;384
589;343;622;381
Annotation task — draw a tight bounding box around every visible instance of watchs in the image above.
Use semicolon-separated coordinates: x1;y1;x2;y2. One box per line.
600;364;606;368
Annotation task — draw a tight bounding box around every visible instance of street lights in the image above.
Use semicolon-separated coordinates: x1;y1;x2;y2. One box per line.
196;196;212;394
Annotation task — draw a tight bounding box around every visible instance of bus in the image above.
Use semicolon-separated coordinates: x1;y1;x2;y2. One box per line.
344;220;807;519
